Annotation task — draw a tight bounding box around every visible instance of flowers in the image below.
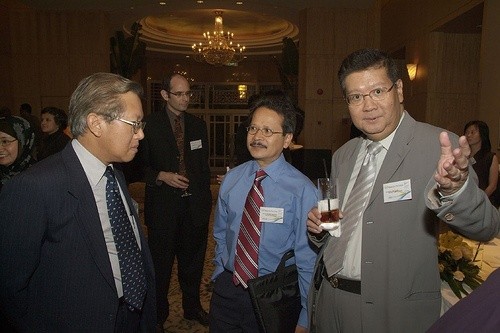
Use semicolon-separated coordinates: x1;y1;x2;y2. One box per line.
438;231;486;300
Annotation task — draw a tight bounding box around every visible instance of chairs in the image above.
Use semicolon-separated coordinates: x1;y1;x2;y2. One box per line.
290;148;333;181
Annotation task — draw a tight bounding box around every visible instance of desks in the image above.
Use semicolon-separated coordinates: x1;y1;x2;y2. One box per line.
437;231;500;318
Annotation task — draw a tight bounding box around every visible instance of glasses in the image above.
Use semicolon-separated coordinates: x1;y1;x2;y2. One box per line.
346;79;399;106
166;90;193;98
97;112;146;134
245;126;290;137
0;139;18;147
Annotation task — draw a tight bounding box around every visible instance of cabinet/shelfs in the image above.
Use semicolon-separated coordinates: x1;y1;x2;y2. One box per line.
296;7;372;151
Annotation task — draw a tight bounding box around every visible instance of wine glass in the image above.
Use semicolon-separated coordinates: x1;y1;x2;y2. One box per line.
176;170;192;197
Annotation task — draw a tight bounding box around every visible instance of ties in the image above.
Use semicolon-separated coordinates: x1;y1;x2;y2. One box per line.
323;143;383;278
103;165;147;310
173;116;189;191
232;168;267;289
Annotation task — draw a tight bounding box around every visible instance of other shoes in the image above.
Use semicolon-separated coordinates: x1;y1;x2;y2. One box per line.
184;309;210;326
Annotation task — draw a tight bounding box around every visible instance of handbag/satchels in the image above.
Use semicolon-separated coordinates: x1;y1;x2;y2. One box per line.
247;250;302;333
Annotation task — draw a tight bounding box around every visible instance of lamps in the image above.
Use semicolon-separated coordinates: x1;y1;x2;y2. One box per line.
145;71;152;81
175;70;201;98
406;63;417;81
191;11;247;69
229;67;252;100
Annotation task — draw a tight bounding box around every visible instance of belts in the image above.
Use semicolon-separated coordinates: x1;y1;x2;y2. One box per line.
322;267;361;295
119;296;125;305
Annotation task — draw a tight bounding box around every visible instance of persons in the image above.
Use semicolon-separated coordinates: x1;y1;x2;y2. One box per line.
205;89;325;333
305;49;500;333
120;73;210;329
463;120;499;210
0;73;159;333
0;103;72;185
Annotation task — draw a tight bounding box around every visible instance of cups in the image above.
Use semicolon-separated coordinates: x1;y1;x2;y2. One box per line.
317;177;340;223
